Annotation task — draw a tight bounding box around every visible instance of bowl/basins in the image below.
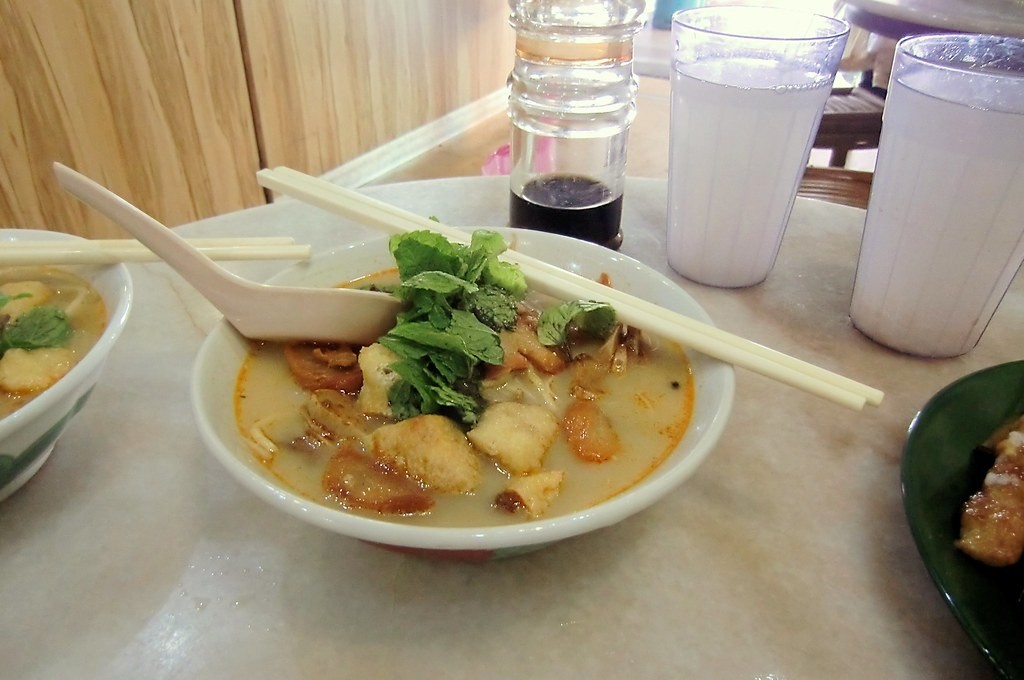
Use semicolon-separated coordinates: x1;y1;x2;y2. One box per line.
0;221;128;501
193;228;736;562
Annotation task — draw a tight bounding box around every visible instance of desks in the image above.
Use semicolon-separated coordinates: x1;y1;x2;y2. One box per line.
849;0;1023;43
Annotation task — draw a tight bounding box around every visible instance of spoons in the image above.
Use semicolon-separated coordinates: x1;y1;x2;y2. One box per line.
54;160;406;344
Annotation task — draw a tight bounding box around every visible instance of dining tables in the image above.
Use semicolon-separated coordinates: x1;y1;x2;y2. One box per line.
1;179;1023;680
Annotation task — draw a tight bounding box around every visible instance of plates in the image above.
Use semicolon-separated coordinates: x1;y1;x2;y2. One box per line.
901;357;1022;677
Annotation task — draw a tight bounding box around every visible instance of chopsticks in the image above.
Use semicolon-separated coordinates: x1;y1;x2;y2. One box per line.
254;162;886;408
0;239;315;266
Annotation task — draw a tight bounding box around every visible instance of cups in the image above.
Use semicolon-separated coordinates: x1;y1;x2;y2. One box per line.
666;2;850;290
848;28;1024;362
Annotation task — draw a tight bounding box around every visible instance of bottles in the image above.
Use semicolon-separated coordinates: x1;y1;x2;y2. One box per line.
502;4;643;255
